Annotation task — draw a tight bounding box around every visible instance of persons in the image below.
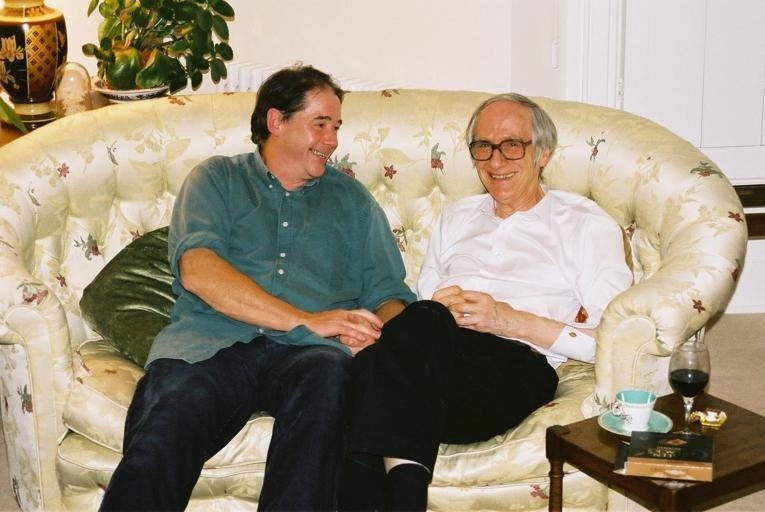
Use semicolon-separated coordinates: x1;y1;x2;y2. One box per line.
101;63;419;512
330;92;634;512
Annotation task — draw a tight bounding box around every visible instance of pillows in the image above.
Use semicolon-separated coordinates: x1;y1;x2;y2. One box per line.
80;225;179;371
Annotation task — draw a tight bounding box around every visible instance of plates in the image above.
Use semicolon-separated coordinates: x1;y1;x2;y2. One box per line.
597;407;673;438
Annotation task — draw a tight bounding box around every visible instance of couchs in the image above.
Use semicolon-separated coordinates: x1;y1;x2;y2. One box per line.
0;91;750;512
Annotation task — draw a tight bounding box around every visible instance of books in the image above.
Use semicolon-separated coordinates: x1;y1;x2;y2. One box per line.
626;431;715;482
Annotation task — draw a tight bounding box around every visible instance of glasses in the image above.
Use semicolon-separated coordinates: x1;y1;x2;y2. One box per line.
469;139;532;161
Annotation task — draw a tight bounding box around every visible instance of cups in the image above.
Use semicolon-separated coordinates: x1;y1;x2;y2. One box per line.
612;389;657;432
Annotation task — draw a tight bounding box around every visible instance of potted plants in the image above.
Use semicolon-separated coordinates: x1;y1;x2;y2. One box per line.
82;0;234;103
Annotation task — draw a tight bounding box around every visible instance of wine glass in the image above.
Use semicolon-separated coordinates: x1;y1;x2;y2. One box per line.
670;342;710;437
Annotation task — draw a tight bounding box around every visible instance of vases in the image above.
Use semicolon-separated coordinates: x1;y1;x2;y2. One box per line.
0;0;68;124
